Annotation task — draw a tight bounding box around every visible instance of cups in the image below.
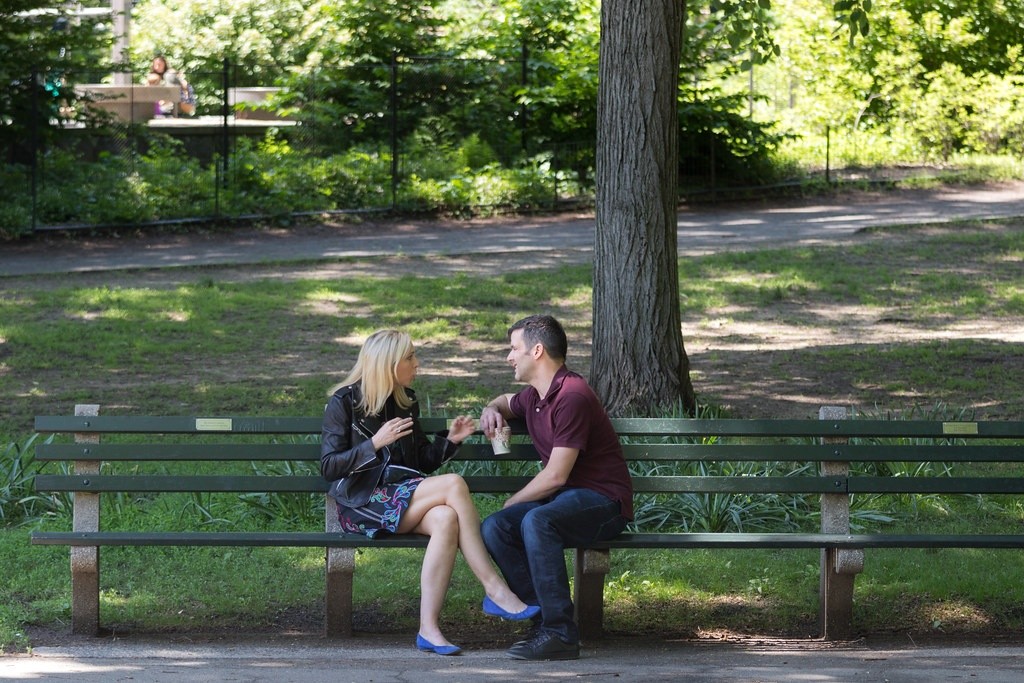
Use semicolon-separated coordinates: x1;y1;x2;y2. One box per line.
490;426;511;455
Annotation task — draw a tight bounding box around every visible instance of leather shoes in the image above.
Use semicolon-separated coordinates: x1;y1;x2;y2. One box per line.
506;629;580;660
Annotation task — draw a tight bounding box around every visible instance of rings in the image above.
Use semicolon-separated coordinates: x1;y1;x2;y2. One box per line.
396;429;400;433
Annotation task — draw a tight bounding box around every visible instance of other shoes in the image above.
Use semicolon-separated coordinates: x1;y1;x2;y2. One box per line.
483;594;541;622
417;634;462;655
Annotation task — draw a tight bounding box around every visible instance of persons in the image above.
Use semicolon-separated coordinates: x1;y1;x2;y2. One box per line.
479;315;635;661
139;56;197;117
320;329;542;656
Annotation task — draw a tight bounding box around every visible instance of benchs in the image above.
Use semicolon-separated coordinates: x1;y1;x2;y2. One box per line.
30;404;1024;640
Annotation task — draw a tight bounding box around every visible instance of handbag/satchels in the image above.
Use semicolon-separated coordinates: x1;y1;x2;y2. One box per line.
381;464;427;484
